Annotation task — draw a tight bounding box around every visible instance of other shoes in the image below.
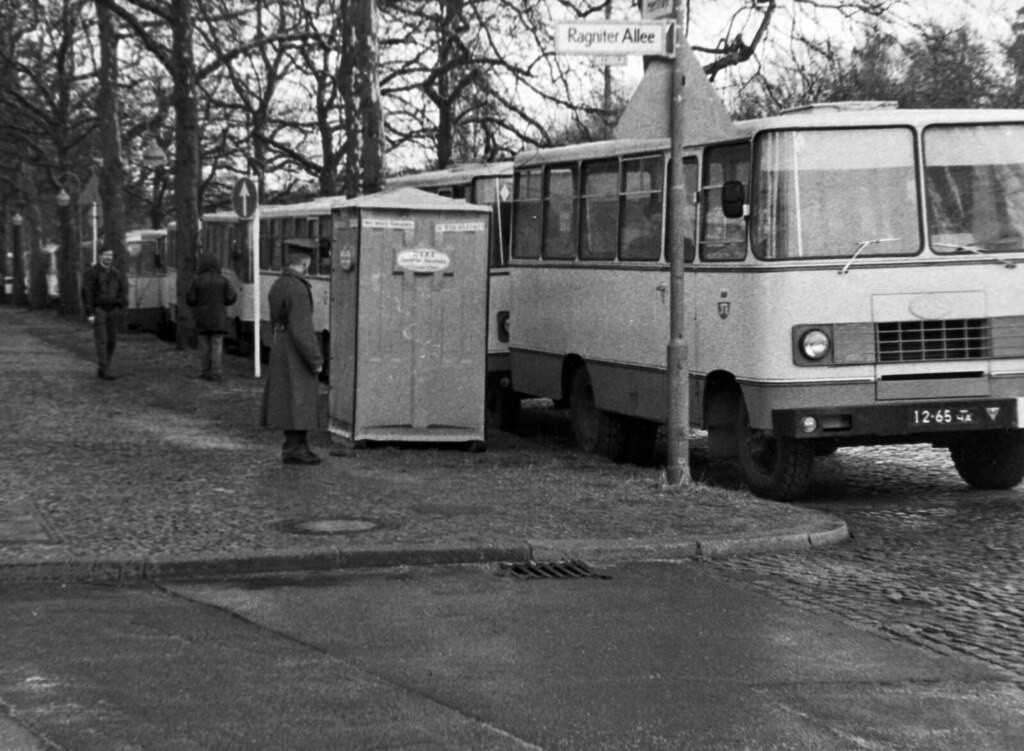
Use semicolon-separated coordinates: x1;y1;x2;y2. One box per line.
282;443;321;464
98;369;115;379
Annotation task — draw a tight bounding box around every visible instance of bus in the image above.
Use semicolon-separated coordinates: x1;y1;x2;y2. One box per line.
505;100;1024;502
0;162;650;391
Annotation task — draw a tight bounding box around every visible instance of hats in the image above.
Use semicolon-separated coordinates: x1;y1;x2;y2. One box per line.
283;238;320;254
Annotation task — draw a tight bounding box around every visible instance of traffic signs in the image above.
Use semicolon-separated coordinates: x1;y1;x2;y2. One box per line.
554;19;677;58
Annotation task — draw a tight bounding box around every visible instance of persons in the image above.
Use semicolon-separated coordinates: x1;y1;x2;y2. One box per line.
259;237;325;465
80;245;130;382
184;251;238;382
622;201;702;262
